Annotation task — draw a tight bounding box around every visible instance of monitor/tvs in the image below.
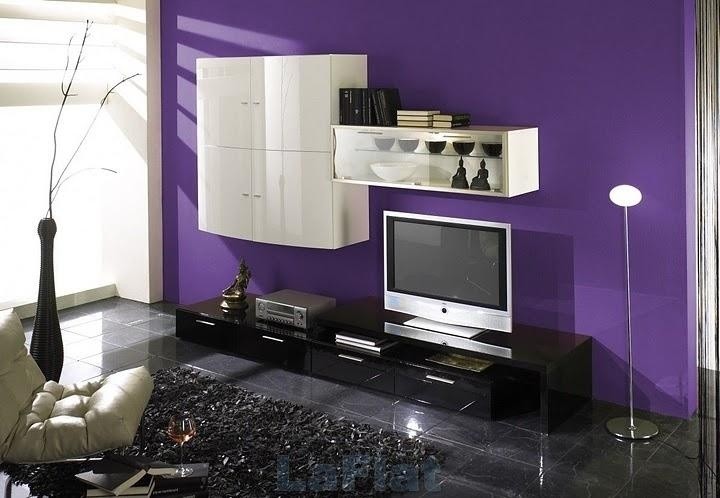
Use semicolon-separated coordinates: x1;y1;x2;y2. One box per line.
384;210;511;339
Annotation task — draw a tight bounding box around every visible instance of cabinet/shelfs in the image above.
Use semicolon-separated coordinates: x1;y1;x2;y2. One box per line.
330;124;540;198
197;52;370;250
175;292;592;436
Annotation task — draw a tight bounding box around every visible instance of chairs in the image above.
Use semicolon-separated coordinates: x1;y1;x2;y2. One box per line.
0;307;154;498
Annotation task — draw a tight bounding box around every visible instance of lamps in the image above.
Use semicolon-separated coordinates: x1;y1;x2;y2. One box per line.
605;185;660;440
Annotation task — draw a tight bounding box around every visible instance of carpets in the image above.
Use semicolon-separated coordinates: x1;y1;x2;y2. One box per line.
3;365;445;498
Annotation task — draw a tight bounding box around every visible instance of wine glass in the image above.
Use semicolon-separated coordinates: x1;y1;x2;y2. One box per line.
167;412;196;478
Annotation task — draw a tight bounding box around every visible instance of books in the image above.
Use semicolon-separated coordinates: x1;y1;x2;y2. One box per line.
148;460;210;489
106;453;182;477
397;111;440;128
75;459;145;496
433;114;474;128
84;487;149;497
339;87;402;128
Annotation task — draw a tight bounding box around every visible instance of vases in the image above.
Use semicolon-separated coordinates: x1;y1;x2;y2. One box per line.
30;215;66;384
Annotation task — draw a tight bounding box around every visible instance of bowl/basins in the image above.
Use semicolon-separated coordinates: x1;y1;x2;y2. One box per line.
370;161;419;182
452;138;475;154
424;139;447;154
481;141;502;157
373;134;395;150
398;137;420;152
